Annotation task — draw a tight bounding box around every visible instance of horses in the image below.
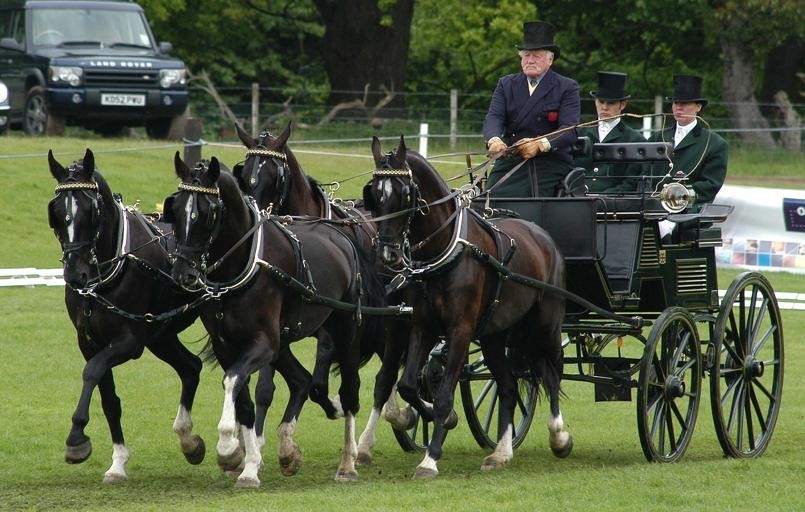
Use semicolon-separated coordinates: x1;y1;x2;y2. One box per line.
232;122;417;478
48;146;207;486
369;134;573;482
168;149;361;490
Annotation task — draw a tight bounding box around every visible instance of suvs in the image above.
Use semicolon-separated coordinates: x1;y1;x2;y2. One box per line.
0;0;190;140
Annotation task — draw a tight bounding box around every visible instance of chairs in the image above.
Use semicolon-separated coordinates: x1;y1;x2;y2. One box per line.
472;136;592;196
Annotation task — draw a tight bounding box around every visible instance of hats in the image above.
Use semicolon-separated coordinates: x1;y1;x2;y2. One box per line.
514;21;560;61
589;70;632;101
665;74;708;102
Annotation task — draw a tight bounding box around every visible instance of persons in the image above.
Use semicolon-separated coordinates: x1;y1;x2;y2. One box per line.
482;19;582;198
568;69;650;196
633;75;729;245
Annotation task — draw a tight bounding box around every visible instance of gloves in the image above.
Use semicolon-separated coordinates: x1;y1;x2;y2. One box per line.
512;137;546;160
487;136;508;161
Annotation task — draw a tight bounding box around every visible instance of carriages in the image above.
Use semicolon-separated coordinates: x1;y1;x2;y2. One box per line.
48;21;784;491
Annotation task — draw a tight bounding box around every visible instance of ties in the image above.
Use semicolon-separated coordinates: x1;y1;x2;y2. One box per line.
530;79;537;87
